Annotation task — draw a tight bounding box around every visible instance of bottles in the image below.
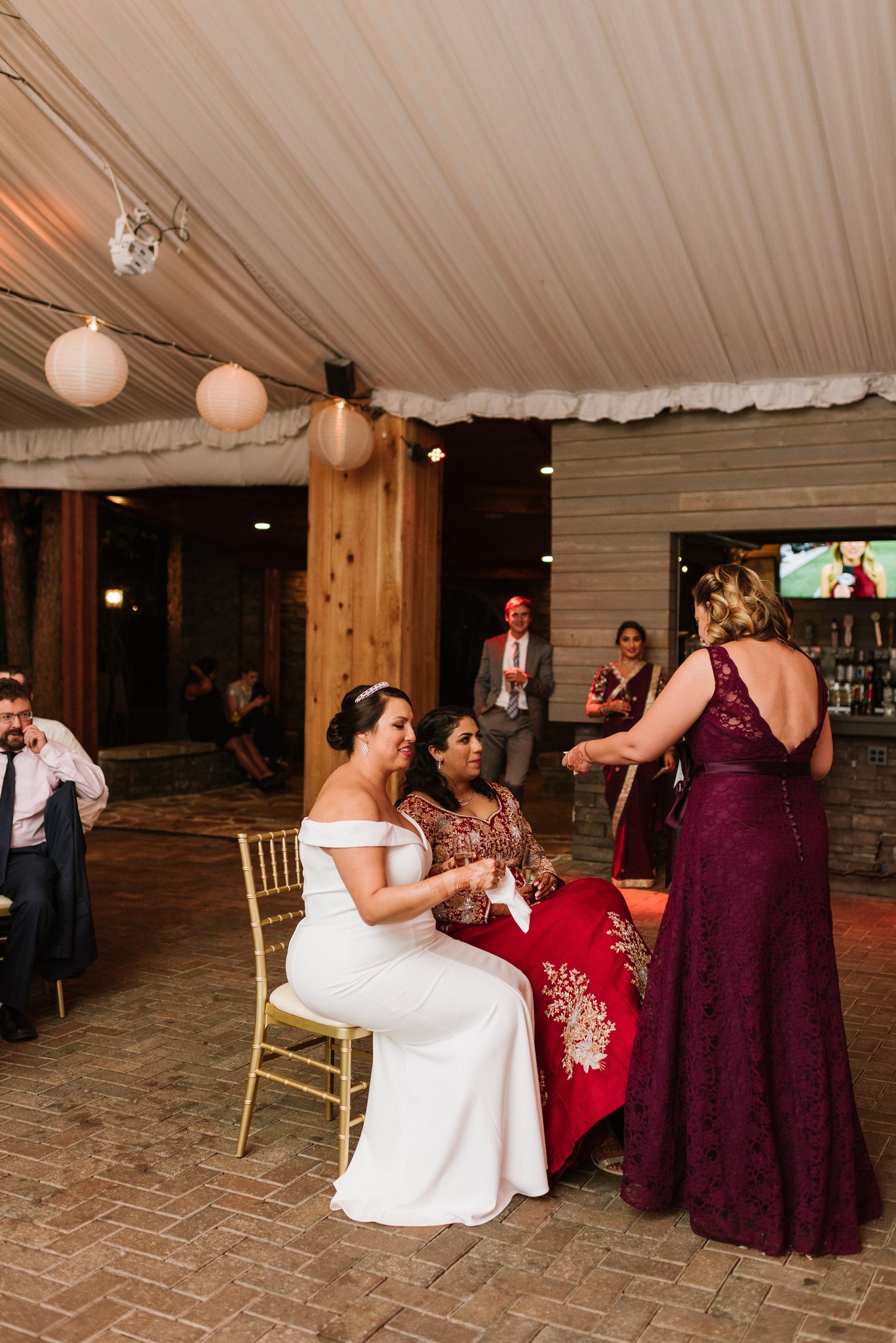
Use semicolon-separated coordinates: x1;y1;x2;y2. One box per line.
797;646;895;717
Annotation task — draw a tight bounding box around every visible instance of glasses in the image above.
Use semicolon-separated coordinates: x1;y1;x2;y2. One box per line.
0;710;33;723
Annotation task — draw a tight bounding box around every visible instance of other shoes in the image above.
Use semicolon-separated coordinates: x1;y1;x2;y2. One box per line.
258;774;286;793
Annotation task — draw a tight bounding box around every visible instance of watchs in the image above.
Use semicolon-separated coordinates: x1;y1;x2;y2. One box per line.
230;711;239;717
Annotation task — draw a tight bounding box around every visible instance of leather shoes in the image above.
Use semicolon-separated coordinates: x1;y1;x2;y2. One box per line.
0;1004;35;1042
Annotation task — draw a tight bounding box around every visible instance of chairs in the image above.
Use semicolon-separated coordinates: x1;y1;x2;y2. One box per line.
235;827;374;1177
0;894;65;1018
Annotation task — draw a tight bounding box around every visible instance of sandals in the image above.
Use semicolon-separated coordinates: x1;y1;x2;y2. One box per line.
589;1152;626;1176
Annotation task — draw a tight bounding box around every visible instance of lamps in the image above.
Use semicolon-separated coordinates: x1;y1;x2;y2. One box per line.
426;444;447;464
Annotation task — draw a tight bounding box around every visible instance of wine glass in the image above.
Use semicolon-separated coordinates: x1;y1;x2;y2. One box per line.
522;844;543;904
452;833;480;910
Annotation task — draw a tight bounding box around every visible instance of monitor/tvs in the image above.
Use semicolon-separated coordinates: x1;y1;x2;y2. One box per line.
774;540;896;602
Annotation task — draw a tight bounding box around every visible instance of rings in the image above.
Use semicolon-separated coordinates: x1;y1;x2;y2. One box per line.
573;770;580;776
526;900;531;905
551;886;556;892
496;876;503;885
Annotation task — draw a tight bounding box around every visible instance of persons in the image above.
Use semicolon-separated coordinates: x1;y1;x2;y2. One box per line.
819;540;888;598
392;701;654;1183
472;595;555;810
0;662;109;838
287;680;551;1227
585;619;676;889
0;677;106;1043
560;563;884;1258
186;655;275;781
227;663;291;770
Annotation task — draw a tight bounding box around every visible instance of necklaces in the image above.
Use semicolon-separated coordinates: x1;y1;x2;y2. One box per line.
457;784;472;806
619;659;639;668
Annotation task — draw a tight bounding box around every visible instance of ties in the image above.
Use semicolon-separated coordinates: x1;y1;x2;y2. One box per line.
0;750;25;887
506;642;520;719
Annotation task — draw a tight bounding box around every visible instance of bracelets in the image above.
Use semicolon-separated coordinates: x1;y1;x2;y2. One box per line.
600;702;609;716
665;746;676;757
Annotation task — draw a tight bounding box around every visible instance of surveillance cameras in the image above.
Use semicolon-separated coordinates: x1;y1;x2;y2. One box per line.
111;196;161;275
